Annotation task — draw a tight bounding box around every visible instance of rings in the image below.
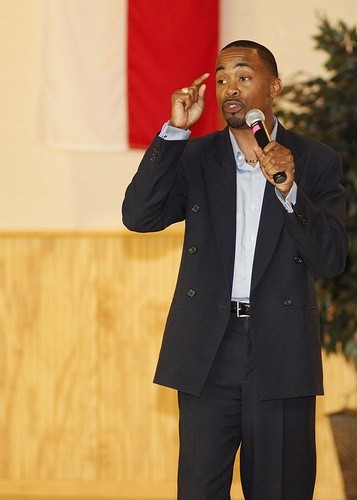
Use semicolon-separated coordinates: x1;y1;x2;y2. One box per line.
181;87;189;94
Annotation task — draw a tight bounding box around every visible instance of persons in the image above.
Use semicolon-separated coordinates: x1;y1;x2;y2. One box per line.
121;40;348;500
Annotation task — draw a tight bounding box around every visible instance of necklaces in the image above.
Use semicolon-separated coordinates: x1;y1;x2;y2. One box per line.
245;160;258;163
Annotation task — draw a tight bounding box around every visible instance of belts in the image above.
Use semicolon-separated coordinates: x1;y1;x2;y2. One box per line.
230;301;251;317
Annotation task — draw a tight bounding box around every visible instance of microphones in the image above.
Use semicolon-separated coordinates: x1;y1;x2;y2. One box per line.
245;109;287;184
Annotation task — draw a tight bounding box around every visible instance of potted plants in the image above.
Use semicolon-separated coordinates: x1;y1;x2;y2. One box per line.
272;19;356;500
